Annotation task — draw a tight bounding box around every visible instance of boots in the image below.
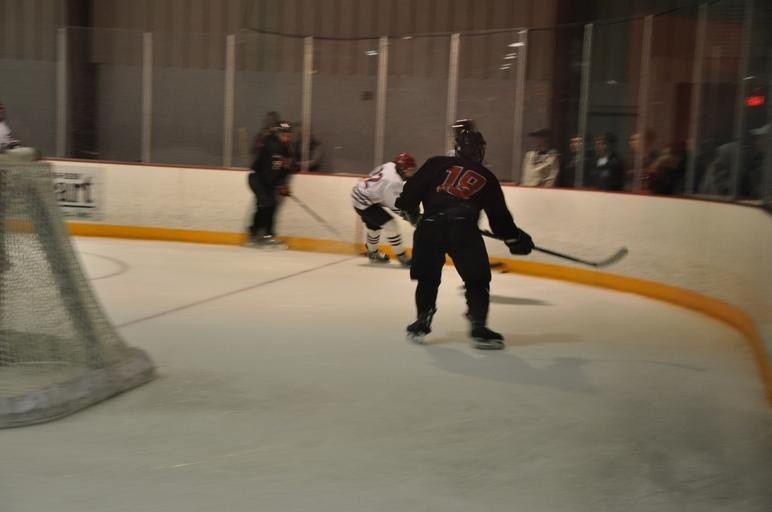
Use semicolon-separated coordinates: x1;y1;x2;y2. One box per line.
250;230;284;246
465;312;504;341
407;306;436;335
396;253;412;265
364;244;390;262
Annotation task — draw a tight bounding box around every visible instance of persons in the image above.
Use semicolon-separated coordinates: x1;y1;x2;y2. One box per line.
394;129;535;340
351;152;419;267
245;120;297;245
521;122;771;204
294;121;324;171
0;103;22;274
249;112;281;156
443;117;489;318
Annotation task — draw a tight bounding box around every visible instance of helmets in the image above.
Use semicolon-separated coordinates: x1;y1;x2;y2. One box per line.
270;120;293;137
394;152;418;181
454;130;486;164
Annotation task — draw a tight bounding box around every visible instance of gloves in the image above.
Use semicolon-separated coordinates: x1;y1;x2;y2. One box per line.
501;228;533;256
276;185;292;197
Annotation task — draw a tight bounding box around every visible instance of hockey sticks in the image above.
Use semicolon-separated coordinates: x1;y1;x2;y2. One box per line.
481;230;628;267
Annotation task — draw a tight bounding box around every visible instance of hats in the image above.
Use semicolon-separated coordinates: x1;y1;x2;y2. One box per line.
451;119;474;128
